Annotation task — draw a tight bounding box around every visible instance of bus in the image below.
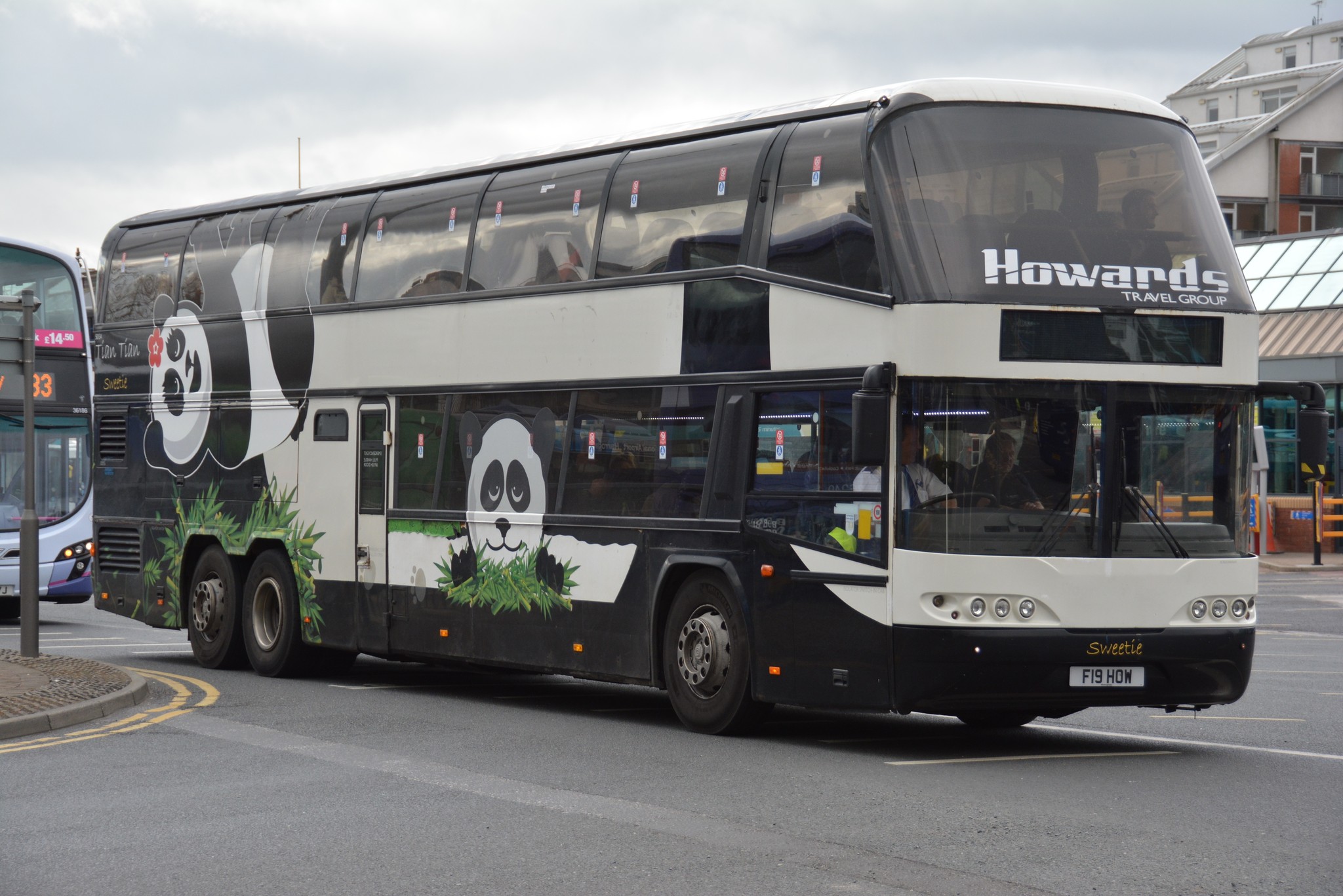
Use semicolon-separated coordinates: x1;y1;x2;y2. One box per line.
90;73;1329;733
0;236;99;620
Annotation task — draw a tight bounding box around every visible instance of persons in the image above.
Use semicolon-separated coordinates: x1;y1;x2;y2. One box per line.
753;447;812;474
852;413;958;558
1100;188;1172;271
969;432;1044;509
570;435;636;514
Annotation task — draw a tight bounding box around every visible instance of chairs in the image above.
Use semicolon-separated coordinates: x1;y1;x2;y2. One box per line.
547;450;862;545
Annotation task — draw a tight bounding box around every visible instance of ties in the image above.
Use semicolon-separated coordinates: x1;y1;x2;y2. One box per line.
901;464;921;509
